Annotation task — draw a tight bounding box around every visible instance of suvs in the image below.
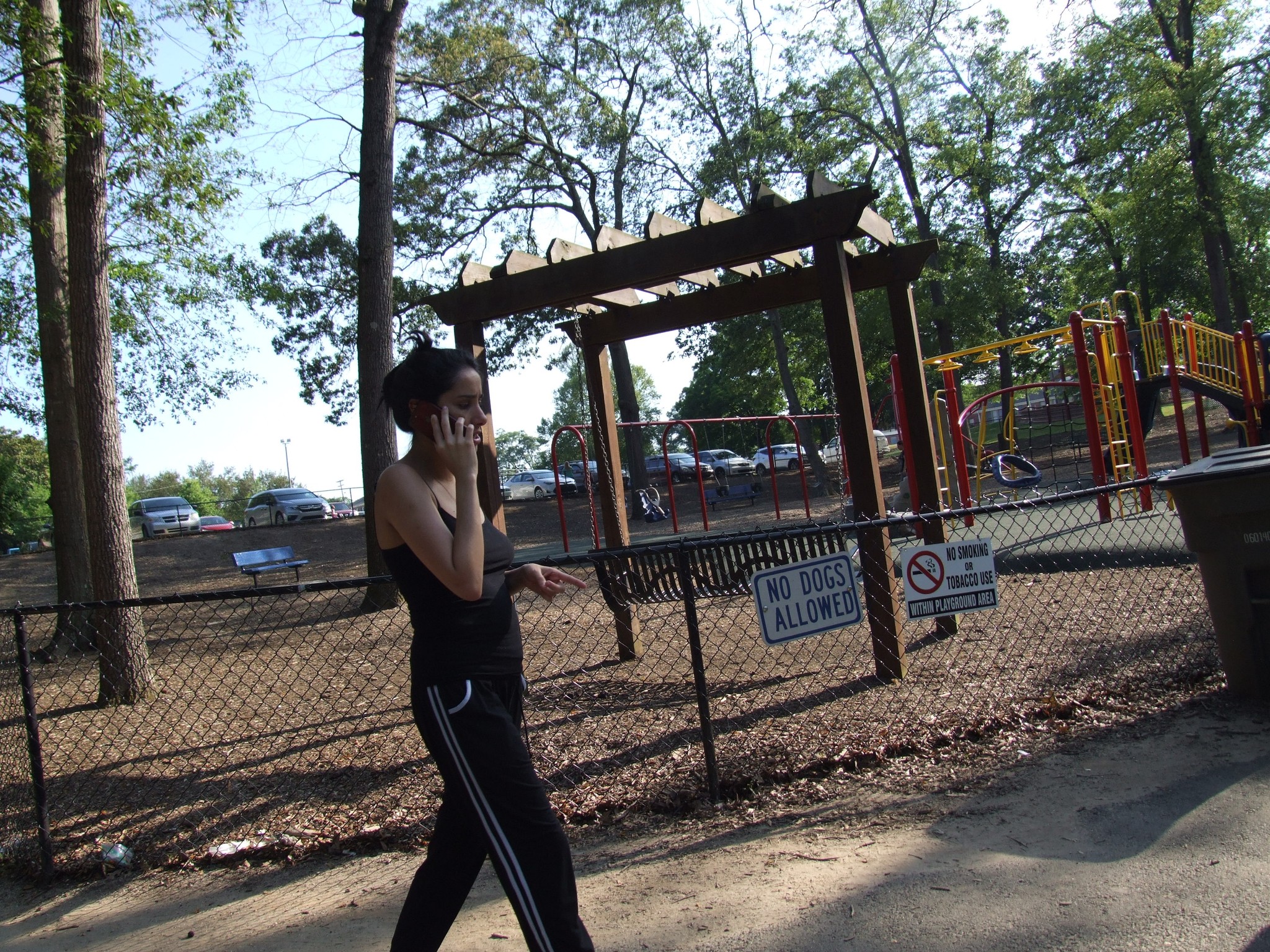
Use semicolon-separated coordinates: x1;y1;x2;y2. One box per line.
645;453;714;484
558;459;630;489
819;429;891;466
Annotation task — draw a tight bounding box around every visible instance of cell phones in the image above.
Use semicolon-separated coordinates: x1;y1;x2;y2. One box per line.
409;401;468;445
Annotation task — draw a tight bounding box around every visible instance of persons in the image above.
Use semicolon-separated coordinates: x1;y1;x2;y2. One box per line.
375;330;593;952
891;441;907;482
331;505;338;518
562;461;574;479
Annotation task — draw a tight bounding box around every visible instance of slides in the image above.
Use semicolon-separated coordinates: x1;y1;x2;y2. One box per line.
1103;391;1159;474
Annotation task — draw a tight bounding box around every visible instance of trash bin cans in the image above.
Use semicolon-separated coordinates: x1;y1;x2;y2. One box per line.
1156;443;1270;721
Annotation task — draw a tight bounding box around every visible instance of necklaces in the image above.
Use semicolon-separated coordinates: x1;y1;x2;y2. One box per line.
434;476;456;502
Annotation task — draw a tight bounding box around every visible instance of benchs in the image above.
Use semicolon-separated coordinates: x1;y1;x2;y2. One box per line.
704;483;761;510
232;546;309;587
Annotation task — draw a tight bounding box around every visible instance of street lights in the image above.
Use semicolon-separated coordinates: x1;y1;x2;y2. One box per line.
280;439;292;488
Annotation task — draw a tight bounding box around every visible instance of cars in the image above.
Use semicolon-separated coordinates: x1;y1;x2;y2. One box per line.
244;487;332;527
128;496;201;542
752;443;827;476
200;516;236;532
328;502;358;518
503;469;579;500
37;514;56;553
688;449;757;479
500;485;513;501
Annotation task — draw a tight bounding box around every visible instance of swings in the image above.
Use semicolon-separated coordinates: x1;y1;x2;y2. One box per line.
587;429;628;508
703;425;730;498
572;304;865;611
629;426;670;523
825;419;851;483
738;422;764;493
795;419;821;489
